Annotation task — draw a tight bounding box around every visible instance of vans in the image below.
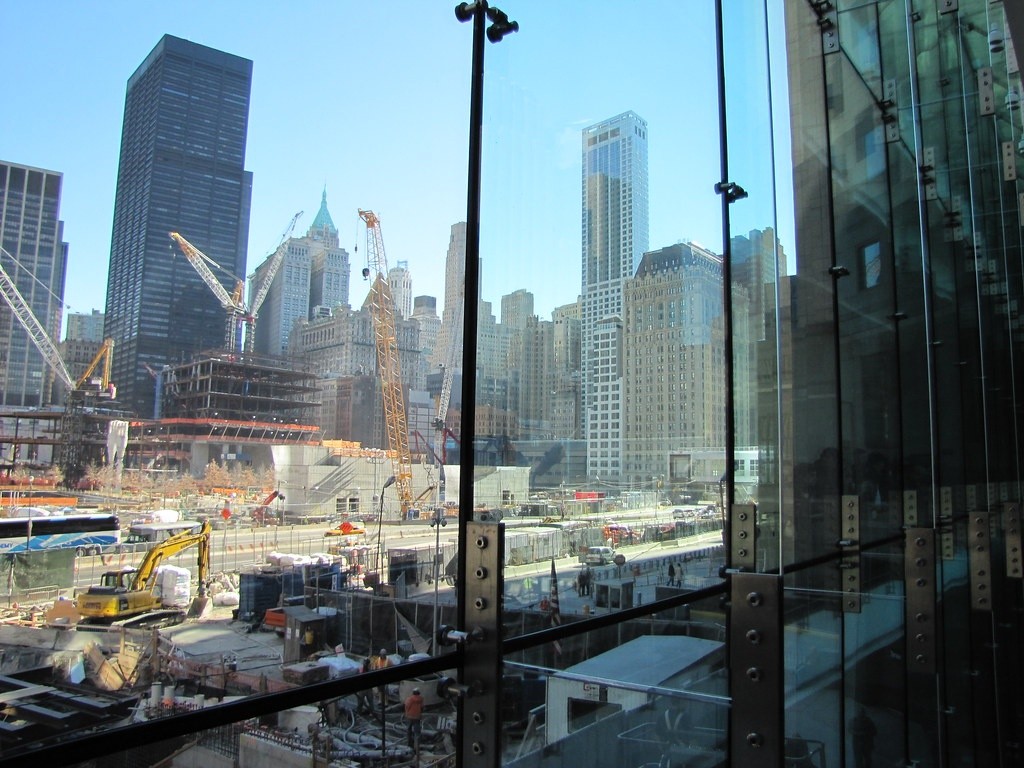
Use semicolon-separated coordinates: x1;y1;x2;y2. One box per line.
585;546;617;566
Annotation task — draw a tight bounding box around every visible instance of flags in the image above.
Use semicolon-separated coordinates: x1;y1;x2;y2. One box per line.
549;558;563;656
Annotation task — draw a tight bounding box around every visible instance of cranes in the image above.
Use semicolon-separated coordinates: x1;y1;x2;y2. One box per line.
0;253;121;402
428;285;465;516
245;209;306;354
169;230;250;355
359;203;432;522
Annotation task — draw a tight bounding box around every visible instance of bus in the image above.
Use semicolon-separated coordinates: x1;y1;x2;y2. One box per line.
0;515;120;557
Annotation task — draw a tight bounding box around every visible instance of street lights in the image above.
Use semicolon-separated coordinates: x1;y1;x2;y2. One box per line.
276;480;287;516
373;476;396;597
454;1;520;524
28;475;35;518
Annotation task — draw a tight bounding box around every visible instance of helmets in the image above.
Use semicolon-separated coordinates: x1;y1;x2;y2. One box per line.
413;688;420;692
380;649;387;654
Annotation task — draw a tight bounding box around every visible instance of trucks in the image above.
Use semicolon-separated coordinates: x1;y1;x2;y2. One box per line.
123;520;203;552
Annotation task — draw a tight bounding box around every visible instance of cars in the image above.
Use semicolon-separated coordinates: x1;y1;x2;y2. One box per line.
672;504;717;520
323;525;364;536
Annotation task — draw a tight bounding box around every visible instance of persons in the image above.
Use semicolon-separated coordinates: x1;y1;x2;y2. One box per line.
539;596;549;611
577;567;597;597
404;687;425;755
357;649;392;716
632;565;640;581
666;561;683;588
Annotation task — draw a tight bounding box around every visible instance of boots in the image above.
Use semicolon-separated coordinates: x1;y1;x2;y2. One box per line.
378;692;388;707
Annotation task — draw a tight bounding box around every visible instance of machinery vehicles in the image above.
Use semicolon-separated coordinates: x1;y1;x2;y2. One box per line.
249;491;285;526
79;519;216;629
